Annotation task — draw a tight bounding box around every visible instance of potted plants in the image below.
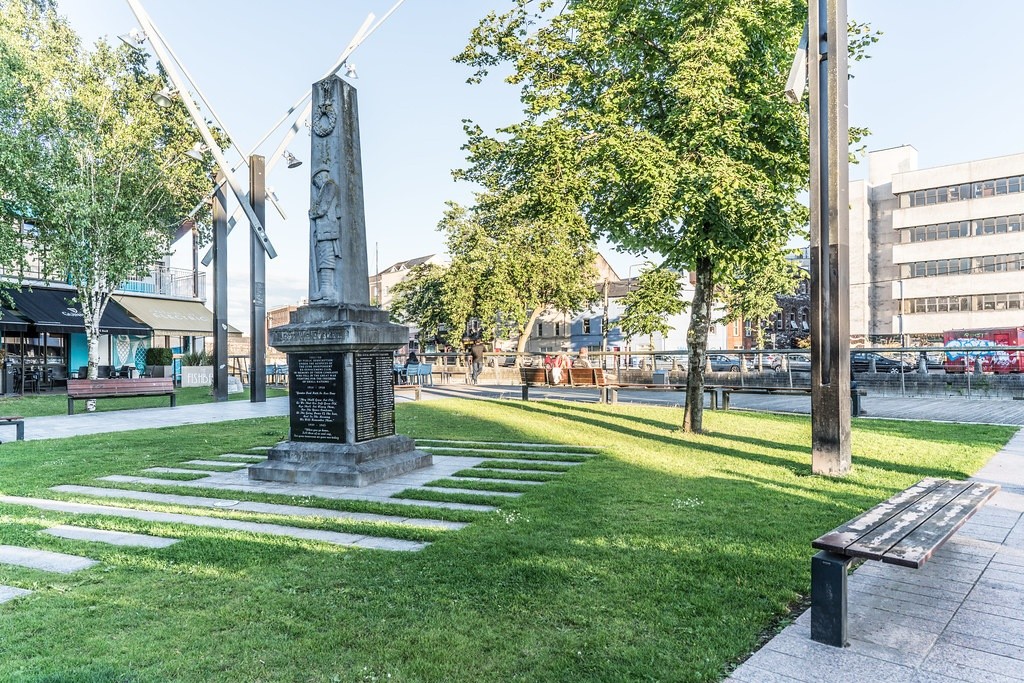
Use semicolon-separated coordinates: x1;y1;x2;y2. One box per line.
144;348;174;378
67;377;180;415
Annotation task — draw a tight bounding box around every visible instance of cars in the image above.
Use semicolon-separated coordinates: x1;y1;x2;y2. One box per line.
489;350;533;367
719;354;754;376
753;354;779;369
850;351;912;374
771;353;811;372
638;355;688;371
915;351;946;365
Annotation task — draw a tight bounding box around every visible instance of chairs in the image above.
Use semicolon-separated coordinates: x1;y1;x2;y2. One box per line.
400;357;433;386
16;366;53;393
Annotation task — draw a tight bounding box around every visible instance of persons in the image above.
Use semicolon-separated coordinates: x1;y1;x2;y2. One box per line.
400;352;418;385
851;370;868;415
308;168;339;302
471;340;488;384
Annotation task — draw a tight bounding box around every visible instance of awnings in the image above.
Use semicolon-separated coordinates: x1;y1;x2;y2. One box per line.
111;295;242;337
0;306;29;332
0;283;151;334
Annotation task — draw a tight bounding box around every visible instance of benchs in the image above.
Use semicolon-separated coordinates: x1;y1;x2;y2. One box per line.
809;475;998;649
0;416;24;441
517;368;868;418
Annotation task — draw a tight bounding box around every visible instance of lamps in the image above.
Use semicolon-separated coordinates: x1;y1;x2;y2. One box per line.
182;141;211;161
282;150;303;169
116;28;150;48
151;76;179;111
344;62;359;80
